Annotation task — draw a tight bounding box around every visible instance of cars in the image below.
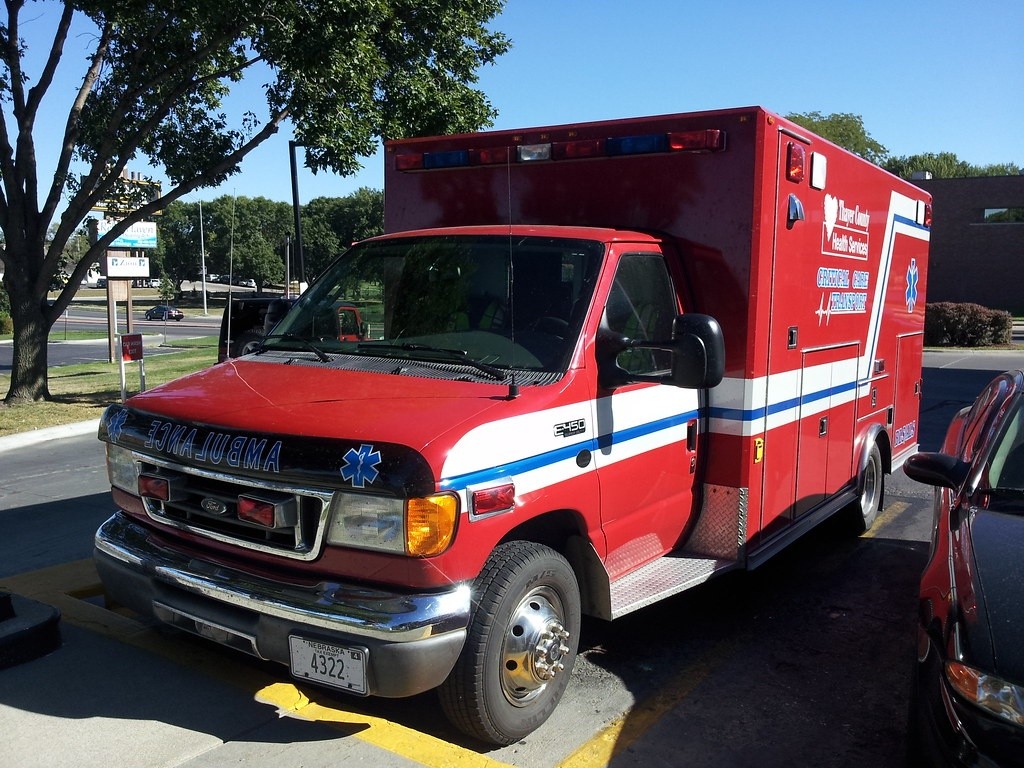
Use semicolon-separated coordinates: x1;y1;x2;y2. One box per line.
144;305;184;322
291;280;298;284
900;364;1024;768
206;274;256;288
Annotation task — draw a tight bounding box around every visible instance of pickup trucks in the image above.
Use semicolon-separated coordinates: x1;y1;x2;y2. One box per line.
148;280;160;288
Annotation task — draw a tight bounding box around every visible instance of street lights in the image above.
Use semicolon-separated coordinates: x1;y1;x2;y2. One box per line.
289;140;312;281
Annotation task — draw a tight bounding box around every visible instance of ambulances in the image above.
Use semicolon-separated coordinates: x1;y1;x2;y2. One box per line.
91;99;933;750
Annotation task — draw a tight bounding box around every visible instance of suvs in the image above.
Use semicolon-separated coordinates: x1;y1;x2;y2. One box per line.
97;279;107;288
217;297;370;363
